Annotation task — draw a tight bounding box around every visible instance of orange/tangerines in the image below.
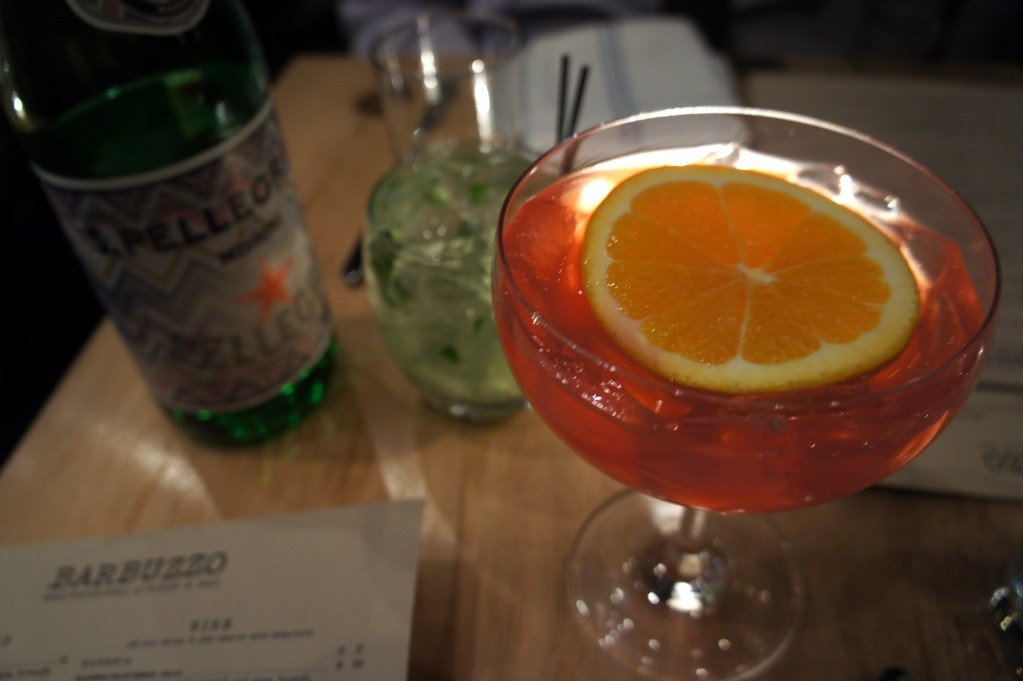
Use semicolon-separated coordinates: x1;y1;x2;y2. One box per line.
578;164;922;397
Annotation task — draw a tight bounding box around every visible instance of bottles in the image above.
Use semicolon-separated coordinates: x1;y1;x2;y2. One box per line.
355;10;567;424
0;0;337;450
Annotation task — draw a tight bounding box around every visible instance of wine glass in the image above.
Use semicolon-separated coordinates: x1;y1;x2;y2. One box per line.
492;107;1004;681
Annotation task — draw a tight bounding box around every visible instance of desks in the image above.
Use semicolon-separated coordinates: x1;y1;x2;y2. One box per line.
0;55;1023;681
